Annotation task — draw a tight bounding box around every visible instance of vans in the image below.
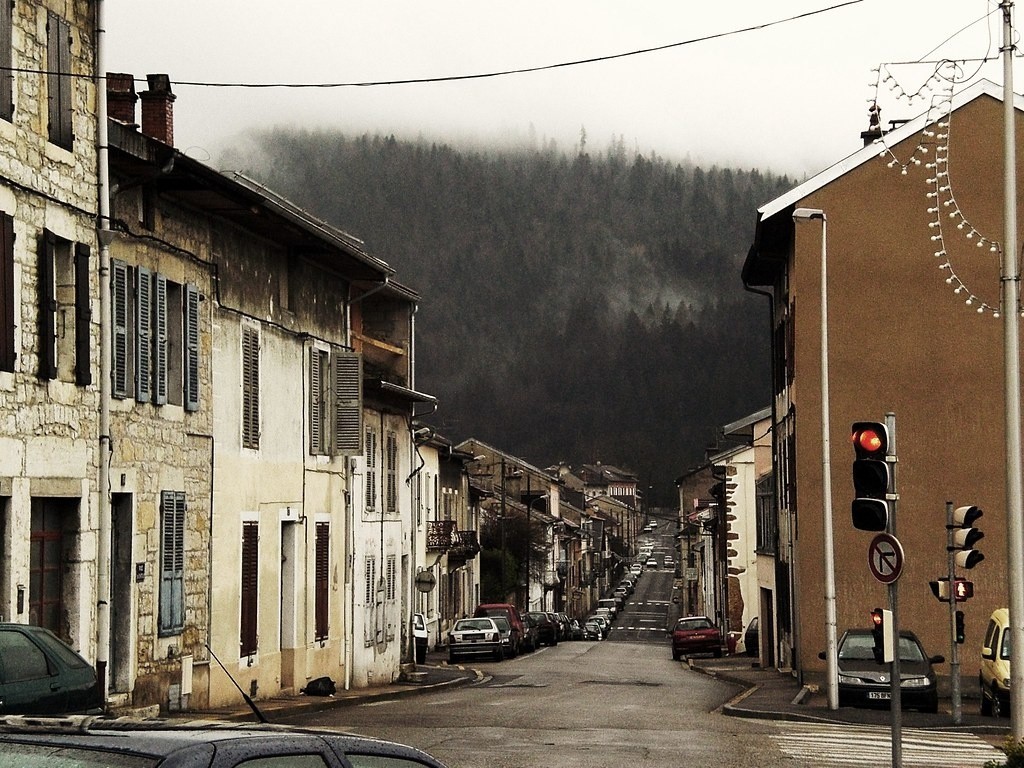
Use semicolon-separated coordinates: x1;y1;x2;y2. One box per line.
979;605;1011;716
472;604;526;654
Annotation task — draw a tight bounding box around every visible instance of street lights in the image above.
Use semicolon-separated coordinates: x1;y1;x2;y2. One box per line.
564;537;583;615
525;495;550;613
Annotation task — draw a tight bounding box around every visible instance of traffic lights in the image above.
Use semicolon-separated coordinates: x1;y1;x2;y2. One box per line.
870;608;894;666
954;579;974;599
929;579;949;603
956;611;965;643
850;420;889;531
955;505;984;569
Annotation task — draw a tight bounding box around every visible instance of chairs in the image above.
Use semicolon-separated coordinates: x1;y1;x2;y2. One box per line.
682;623;689;628
851;645;867;657
899;646;911;659
498;621;506;628
700;622;707;626
481;624;491;628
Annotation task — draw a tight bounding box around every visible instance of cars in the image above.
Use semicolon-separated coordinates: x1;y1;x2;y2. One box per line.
520;613;543;650
650;520;658;527
1;714;448;768
664;555;674;568
644;525;652;533
548;538;655;664
818;627;946;713
647;557;658;567
0;621;104;718
446;618;506;663
486;615;517;656
528;610;559;646
667;614;724;660
